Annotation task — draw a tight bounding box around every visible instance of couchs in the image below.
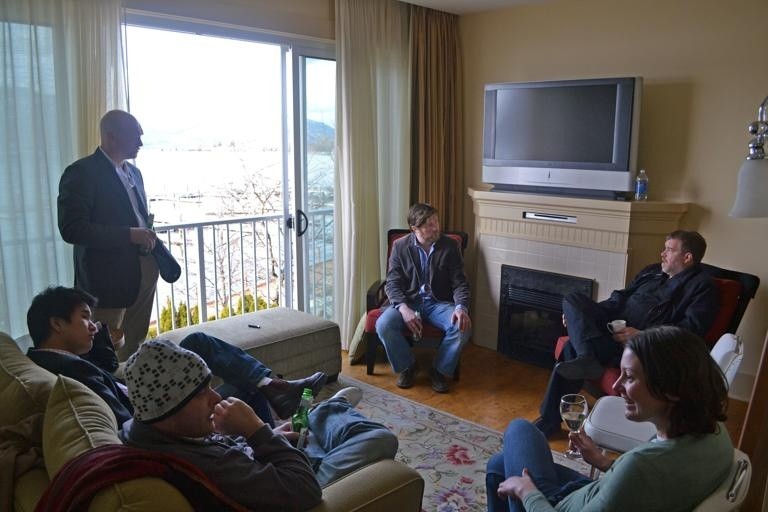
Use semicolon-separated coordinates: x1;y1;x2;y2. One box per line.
0;331;425;512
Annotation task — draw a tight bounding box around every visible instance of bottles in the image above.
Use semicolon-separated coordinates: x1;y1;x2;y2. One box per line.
411;308;422;344
140;213;155;256
288;387;314;448
635;169;649;200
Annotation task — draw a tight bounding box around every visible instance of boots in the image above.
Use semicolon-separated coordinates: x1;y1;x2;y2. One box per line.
258;372;325;421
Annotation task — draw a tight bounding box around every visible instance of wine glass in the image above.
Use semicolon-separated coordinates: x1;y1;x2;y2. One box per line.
560;393;589;460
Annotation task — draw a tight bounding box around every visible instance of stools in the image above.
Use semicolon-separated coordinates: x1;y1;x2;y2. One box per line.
156;307;342;383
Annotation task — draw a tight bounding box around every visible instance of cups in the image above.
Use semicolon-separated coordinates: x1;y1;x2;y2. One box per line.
607;320;627;336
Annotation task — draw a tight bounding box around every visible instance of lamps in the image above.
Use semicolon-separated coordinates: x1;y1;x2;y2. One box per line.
727;96;768;219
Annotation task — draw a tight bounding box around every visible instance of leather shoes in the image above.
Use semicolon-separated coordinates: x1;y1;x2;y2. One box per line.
533;416;562;438
554;355;599;380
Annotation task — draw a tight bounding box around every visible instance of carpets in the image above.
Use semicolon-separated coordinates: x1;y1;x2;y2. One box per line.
272;371;605;512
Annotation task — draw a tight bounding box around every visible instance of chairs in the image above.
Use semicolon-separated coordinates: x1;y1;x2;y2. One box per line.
553;264;761;411
366;229;468;382
693;446;752;512
584;333;743;480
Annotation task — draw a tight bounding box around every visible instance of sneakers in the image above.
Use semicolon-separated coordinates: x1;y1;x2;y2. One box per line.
332;385;363;409
428;369;448;392
397;371;413;389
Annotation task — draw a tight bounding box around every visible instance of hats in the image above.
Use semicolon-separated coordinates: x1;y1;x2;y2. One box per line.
124;339;215;423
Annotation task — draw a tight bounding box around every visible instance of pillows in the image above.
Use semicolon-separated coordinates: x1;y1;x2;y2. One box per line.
0;331;56;440
44;374;196;512
349;311;366;366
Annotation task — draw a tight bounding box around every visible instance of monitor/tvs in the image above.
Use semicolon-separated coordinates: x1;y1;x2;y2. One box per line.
481;76;643;201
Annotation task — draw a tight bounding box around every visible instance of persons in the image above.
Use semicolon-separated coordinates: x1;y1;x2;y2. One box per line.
117;337;400;510
484;325;736;512
374;203;473;394
55;109;161;365
26;284;329;430
533;228;721;438
